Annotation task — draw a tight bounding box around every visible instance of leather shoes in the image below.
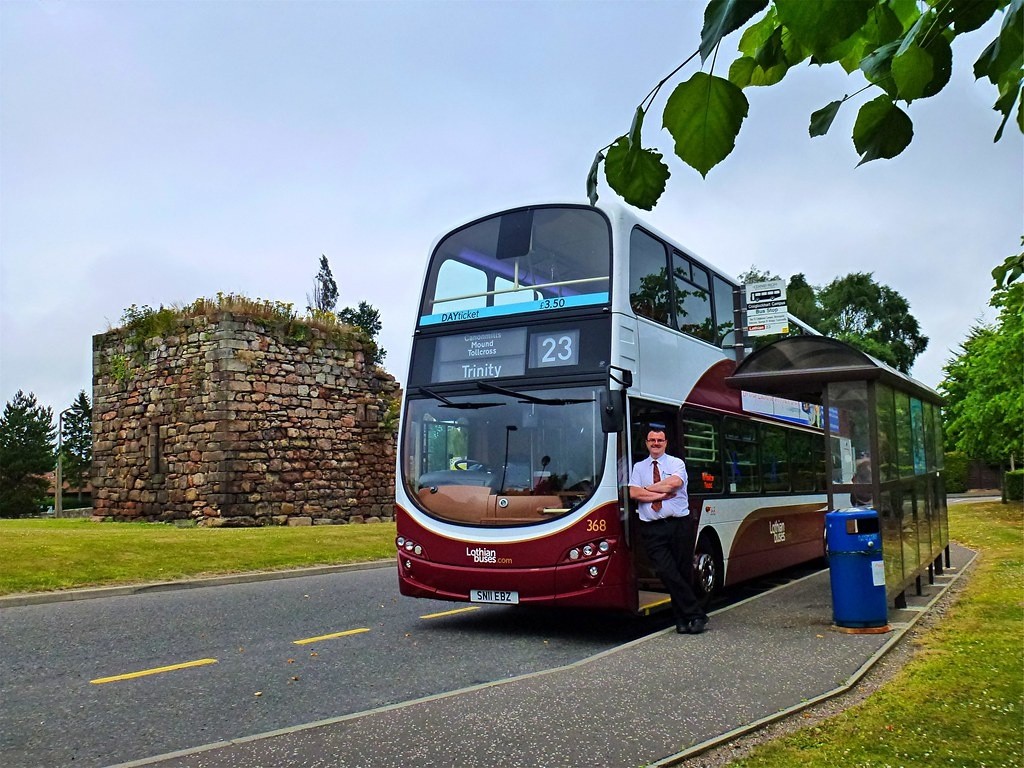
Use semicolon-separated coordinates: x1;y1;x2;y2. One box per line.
689;614;709;633
676;619;690;633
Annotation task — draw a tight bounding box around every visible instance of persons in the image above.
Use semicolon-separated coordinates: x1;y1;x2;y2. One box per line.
628;429;708;633
852;453;873;507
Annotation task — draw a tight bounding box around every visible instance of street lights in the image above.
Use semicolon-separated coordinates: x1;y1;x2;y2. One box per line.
55;407;81;517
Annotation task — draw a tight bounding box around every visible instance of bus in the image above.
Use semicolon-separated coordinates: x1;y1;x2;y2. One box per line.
394;203;855;617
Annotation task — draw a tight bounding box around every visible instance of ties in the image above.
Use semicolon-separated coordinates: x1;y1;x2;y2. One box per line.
651;461;663;512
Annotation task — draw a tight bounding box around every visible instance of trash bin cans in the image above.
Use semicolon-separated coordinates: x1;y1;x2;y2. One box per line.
824;508;891;635
